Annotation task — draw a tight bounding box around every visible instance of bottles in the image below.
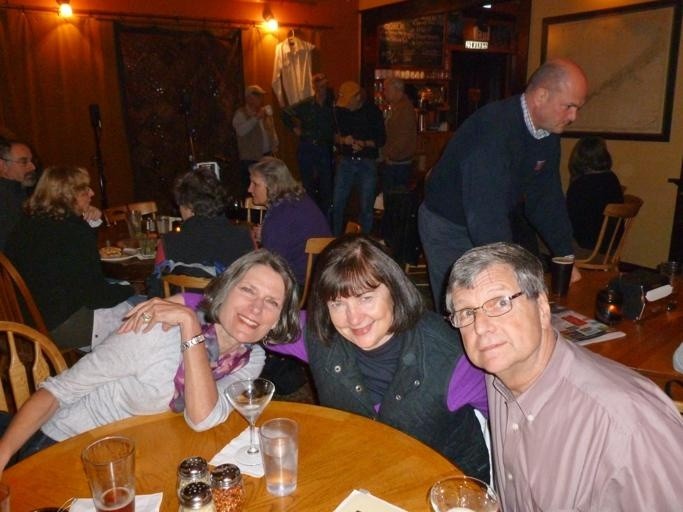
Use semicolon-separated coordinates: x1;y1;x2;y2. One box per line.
594;287;623;326
177;482;217;512
210;463;245;512
146;216;157;234
174;456;211;502
372;78;448;133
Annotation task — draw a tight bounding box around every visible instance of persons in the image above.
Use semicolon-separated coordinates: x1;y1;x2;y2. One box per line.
416;60;589;316
164;238;489;473
279;73;335;217
332;81;385;235
444;241;683;512
232;86;279;224
567;137;623;260
379;76;420;267
0;139;333;472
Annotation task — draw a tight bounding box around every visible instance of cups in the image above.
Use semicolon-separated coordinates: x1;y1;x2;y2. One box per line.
0;481;11;512
429;476;499;512
660;261;682;311
125;210;142;238
157;219;169;238
80;434;138;512
258;417;300;496
550;256;574;297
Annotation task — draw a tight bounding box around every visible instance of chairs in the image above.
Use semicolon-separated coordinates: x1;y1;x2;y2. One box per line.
0;194;642;416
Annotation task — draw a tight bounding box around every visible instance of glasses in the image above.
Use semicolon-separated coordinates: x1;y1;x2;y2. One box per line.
0;157;36;166
445;291;524;328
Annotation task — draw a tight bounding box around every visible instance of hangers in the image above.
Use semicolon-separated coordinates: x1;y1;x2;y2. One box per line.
280;26;309;44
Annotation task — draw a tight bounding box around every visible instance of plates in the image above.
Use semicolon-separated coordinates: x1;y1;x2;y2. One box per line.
99;248;136;262
134;248;157;260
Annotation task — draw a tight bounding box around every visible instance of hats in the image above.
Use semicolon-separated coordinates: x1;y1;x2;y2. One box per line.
247;86;267;95
336;82;359;107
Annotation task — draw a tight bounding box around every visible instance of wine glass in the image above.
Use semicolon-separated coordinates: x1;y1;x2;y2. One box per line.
223;378;278;466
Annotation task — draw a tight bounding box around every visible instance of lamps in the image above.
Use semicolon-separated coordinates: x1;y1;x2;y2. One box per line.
58;0;72;17
264;12;277;29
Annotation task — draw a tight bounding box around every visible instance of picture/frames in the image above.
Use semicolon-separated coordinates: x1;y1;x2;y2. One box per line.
539;0;683;143
